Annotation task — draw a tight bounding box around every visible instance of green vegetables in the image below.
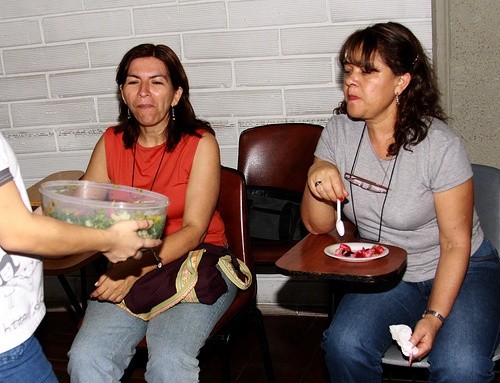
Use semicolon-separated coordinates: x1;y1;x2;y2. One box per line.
43;185;165;239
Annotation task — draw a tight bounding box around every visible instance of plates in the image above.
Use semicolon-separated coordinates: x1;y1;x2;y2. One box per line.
324;243;390;262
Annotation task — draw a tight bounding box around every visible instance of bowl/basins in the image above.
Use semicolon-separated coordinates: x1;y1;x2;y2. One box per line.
38;179;170;251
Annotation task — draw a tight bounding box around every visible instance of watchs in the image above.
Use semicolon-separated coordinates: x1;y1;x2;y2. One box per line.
151;250;163;269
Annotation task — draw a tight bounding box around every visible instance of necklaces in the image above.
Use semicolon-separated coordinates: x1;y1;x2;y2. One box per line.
131;140;167;200
350;123;398;242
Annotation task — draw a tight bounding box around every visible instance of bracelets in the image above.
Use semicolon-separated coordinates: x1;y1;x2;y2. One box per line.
422;310;444;321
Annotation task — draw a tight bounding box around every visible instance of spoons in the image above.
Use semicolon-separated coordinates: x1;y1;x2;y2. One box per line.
335;198;345;236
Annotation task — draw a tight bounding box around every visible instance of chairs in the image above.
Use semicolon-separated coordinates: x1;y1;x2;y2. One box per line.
25;123;500;383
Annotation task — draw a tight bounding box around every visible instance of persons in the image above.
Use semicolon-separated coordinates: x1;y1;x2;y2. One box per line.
0;132;163;383
67;43;238;383
299;21;500;383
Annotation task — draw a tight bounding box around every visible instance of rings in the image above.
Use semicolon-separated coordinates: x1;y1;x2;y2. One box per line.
419;357;420;360
315;181;321;186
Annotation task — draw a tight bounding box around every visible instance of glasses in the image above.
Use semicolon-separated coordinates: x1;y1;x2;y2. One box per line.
344;172;389;194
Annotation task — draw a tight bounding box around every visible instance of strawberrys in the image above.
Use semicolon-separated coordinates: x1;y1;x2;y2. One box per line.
335;243;385;257
